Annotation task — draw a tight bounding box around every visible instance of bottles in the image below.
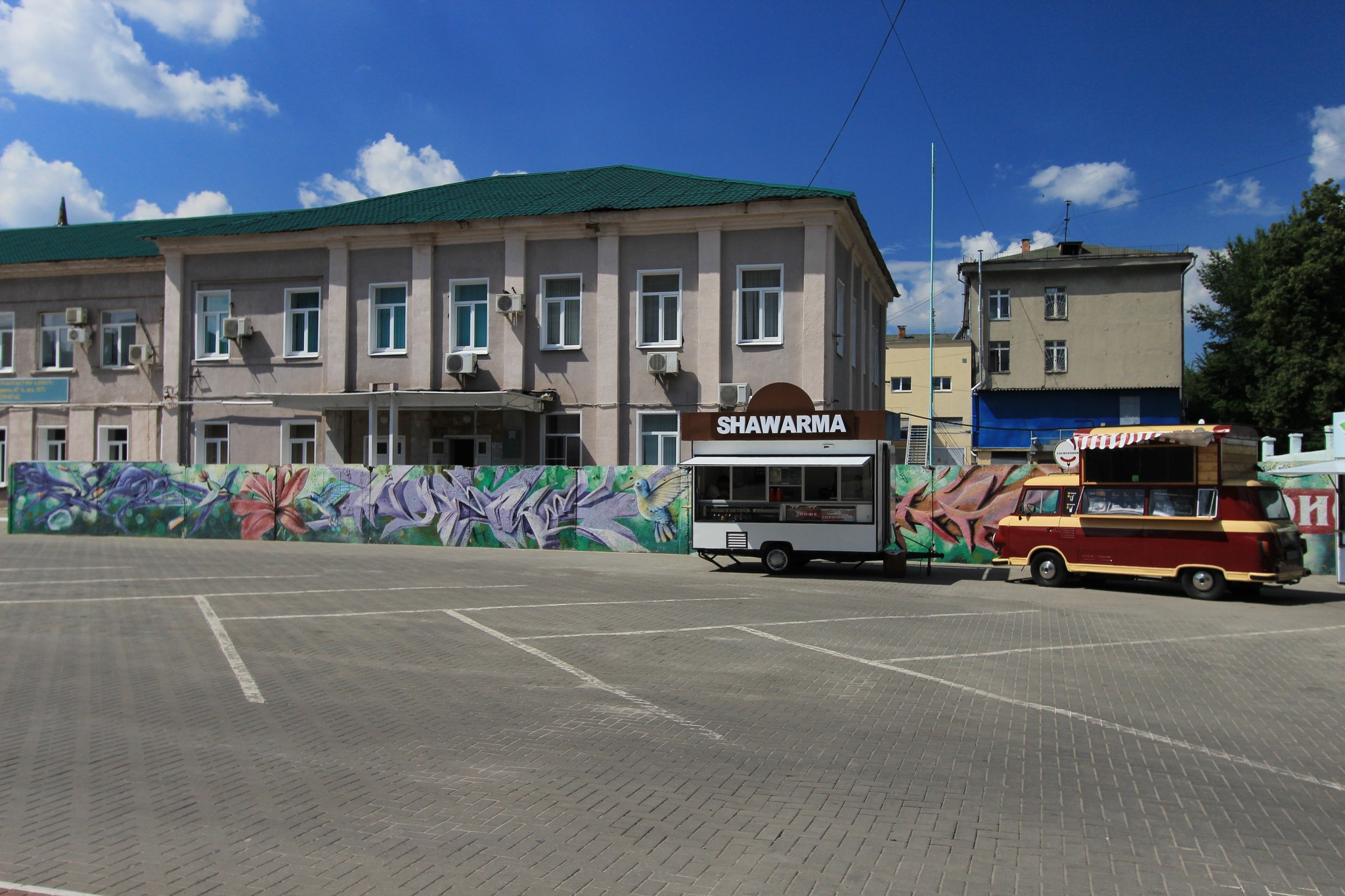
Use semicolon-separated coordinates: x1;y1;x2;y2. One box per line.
713;509;743;522
769;488;774;502
776;487;781;502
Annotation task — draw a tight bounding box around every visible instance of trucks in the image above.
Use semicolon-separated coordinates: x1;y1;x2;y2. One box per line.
990;416;1312;601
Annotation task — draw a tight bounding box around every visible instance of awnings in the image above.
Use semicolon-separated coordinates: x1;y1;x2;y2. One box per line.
1266;460;1345;475
678;456;875;468
1067;427;1216;452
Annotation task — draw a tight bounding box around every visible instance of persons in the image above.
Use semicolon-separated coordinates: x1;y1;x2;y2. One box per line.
707;476;730;500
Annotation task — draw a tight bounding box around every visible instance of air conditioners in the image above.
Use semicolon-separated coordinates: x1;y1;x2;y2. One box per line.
129;344;152;365
445;353;477;373
718;383;752;405
67;328;92;345
647;351;679;375
223;317;252;339
66;307;87;324
496;294;523;313
